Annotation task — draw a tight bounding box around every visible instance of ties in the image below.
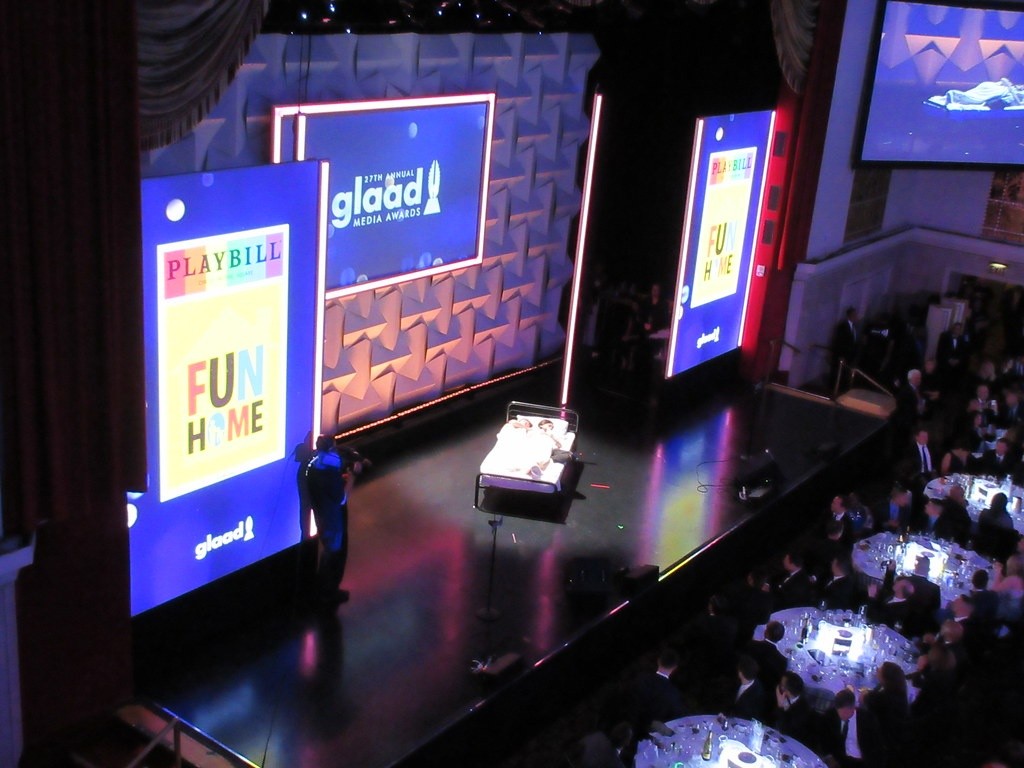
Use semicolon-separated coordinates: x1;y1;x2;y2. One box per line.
997;456;1000;461
851;324;854;334
922;446;929;474
842;719;850;743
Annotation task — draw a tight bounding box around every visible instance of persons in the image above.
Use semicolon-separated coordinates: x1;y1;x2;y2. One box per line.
934;485;971;549
972;494;1018;564
858;558;914;632
871;479;910;536
814;520;848;578
1016;538;1024;567
862;281;1024;491
830;305;858;388
577;722;632;768
687;594;738;682
642;282;666;331
307;434;351;602
894;461;939;530
724;566;1024;767
509;418;581;477
630;645;678;728
991;556;1024;621
807;495;854;546
777;549;814;609
959;568;999;640
818;555;860;614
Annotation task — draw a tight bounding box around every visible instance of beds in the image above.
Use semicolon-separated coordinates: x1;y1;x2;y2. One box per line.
474;401;579;524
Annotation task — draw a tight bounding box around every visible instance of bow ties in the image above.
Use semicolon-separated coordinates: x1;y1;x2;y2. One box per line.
787;698;792;707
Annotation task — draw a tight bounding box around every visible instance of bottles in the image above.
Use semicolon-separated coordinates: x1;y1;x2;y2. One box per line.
702;730;714;760
801;619;809;643
905;526;910;541
899;535;904;555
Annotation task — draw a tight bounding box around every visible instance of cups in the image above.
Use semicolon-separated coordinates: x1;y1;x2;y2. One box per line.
793;608;912;677
768;738;779;748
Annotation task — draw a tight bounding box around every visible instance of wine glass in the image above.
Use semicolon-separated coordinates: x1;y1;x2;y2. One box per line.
894;621;902;636
692;723;699;737
722;721;728;734
818;599;827;615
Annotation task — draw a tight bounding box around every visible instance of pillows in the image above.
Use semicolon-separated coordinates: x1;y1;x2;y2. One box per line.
516;414;569;437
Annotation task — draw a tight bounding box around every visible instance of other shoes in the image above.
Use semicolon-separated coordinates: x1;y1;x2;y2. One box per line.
333;590;350;602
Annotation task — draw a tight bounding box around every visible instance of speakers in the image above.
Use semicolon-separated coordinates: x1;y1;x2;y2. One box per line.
477;652;528;697
623;565;659;596
564;560;612;601
737;444;778;492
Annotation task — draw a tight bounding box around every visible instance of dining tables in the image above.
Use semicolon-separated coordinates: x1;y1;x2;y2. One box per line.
925;428;1024;541
849;531;997;622
632;714;829;768
754;606;923;716
